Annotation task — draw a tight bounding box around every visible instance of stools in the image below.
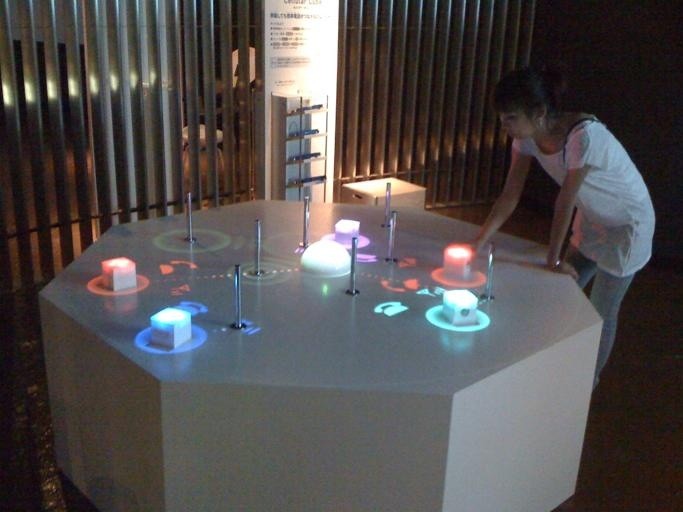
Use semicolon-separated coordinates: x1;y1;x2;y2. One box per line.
340;176;426;210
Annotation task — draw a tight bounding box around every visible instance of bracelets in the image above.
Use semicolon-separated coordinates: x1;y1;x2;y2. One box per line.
550;260;560;270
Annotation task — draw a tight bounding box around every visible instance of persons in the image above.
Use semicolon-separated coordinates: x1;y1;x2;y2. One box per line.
466;68;656;393
